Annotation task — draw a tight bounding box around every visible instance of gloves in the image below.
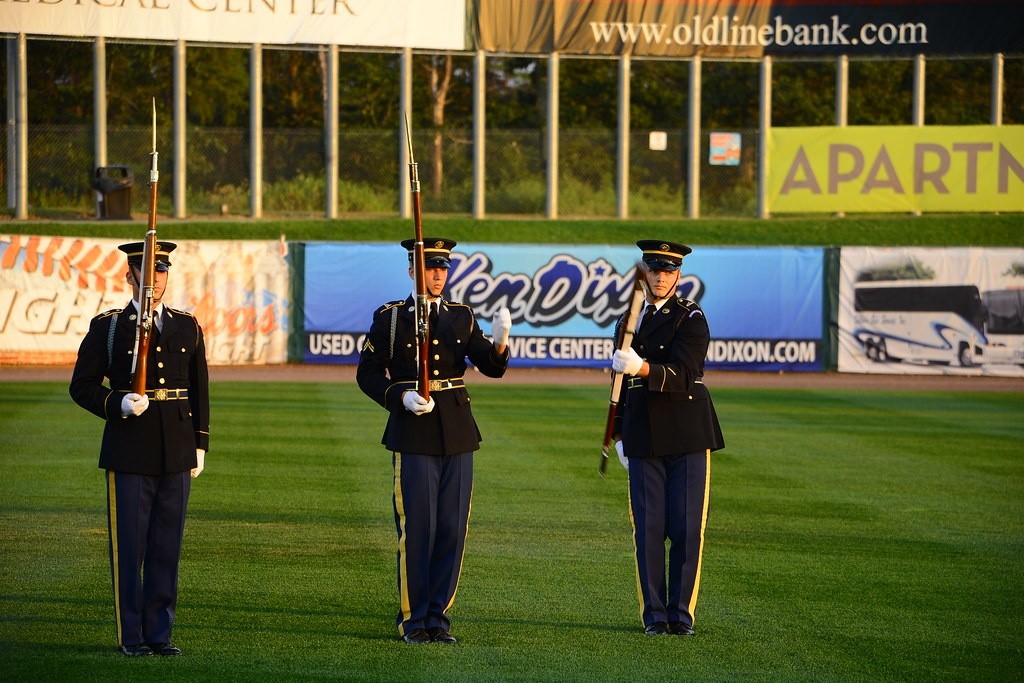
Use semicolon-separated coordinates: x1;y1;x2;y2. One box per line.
491;307;512;346
190;448;206;478
403;391;435;416
120;393;149;417
615;440;629;471
611;346;643;377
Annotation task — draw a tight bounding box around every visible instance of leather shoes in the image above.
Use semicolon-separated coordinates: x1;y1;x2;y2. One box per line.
645;621;668;635
427;629;457;643
669;623;695;636
154;643;182;656
121;643;155;657
402;628;430;643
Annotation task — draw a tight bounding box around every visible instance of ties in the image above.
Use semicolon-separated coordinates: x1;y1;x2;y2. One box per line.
638;304;657;334
150;310;161;348
428;302;439;338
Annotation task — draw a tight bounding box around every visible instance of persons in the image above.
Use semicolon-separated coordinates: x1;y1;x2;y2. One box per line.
610;239;726;636
70;241;210;658
355;237;513;645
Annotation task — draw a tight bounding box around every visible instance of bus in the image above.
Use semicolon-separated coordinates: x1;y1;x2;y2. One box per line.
853;280;1023;368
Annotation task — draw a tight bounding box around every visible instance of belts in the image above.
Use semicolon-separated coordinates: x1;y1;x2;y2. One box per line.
395;377;466;391
117;388;189;401
628;375;703;389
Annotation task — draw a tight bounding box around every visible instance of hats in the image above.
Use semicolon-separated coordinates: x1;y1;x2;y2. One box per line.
118;241;177;271
400;238;457;268
637;240;692;271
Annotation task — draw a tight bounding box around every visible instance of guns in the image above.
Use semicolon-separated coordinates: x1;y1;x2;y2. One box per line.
597;261;649;478
403;109;431;403
131;96;159;402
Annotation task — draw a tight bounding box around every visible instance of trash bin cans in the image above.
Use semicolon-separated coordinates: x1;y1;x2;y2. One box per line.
93;164;134;221
